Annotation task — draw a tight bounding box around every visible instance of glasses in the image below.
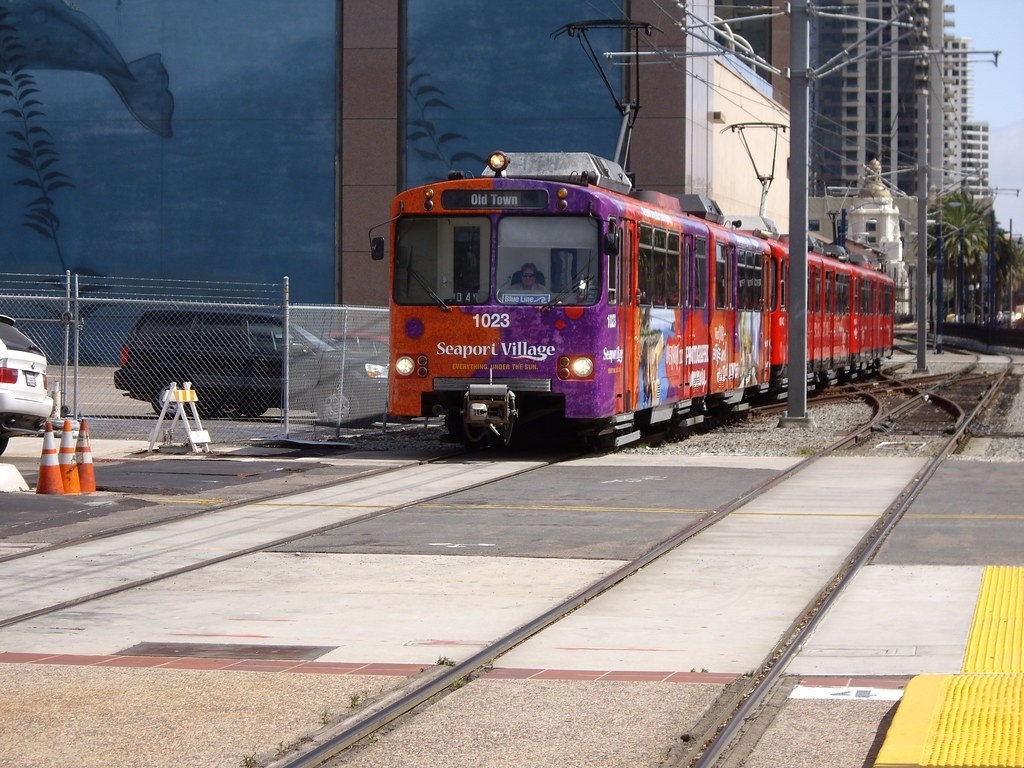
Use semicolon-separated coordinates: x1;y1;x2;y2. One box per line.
522;272;535;278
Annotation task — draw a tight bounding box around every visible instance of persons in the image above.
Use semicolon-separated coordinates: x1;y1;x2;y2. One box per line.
508;263;548;291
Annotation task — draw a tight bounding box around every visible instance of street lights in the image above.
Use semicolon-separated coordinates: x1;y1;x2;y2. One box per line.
74;418;96;493
58;420;82;496
35;422;65;496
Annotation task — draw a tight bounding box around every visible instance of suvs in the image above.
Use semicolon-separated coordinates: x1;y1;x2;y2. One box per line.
113;305;389;432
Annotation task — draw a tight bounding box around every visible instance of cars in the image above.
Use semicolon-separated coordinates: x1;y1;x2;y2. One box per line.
0;314;54;455
972;307;1024;327
320;328;389;368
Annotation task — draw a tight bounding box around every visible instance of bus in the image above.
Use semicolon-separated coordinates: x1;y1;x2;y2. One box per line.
363;18;896;456
